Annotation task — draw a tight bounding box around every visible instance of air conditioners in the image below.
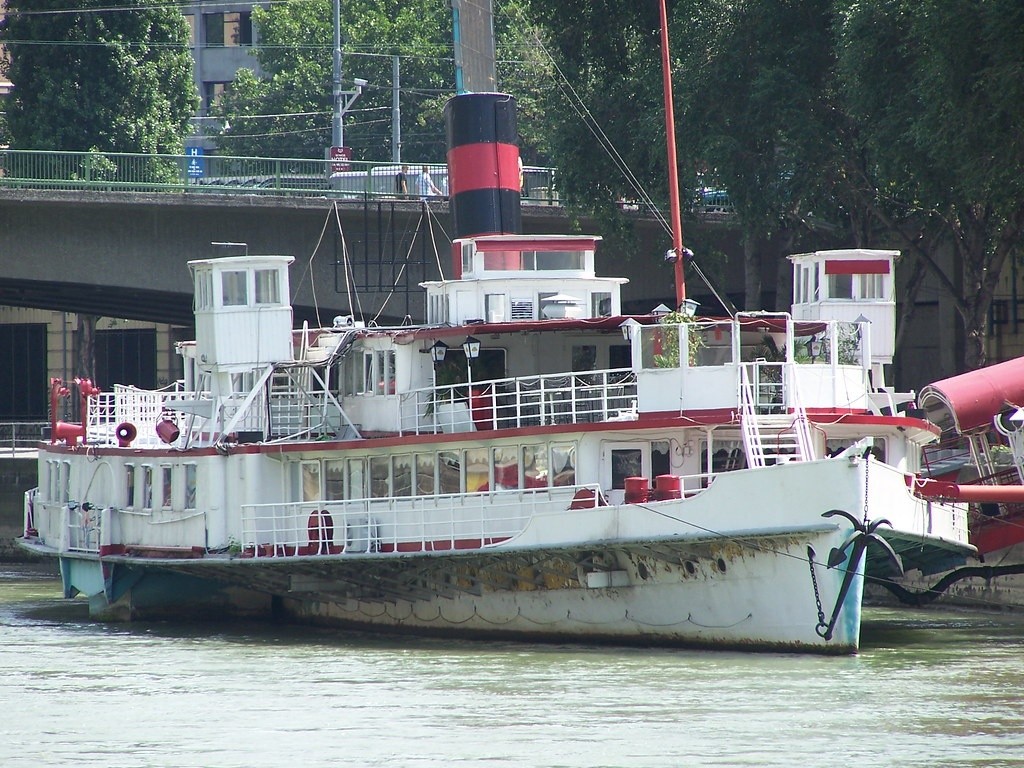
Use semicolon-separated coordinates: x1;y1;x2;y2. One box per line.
346;518;380;552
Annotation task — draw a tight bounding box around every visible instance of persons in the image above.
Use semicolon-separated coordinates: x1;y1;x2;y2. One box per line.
396;166;409;200
415;166;442;200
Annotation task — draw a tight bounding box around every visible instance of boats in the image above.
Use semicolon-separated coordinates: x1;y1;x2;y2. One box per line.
15;2;983;652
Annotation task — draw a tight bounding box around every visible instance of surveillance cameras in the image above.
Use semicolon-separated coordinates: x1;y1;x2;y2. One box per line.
354;78;369;86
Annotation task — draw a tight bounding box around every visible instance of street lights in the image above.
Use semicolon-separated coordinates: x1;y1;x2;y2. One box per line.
331;77;367;165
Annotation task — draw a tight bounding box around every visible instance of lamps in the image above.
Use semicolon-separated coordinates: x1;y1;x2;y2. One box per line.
652;304;672;323
678;299;701;318
683;247;694;262
664;247;679;264
618;317;642;343
805;336;823;364
460;335;481;366
419;339;449;367
